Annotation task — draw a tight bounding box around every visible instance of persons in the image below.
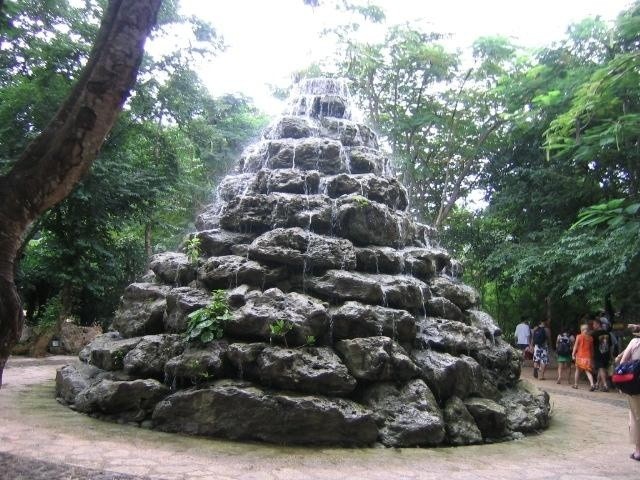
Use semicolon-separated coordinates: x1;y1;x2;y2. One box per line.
509;310;640;464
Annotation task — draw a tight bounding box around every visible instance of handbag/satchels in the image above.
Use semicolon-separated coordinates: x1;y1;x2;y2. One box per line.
611;358;640;395
